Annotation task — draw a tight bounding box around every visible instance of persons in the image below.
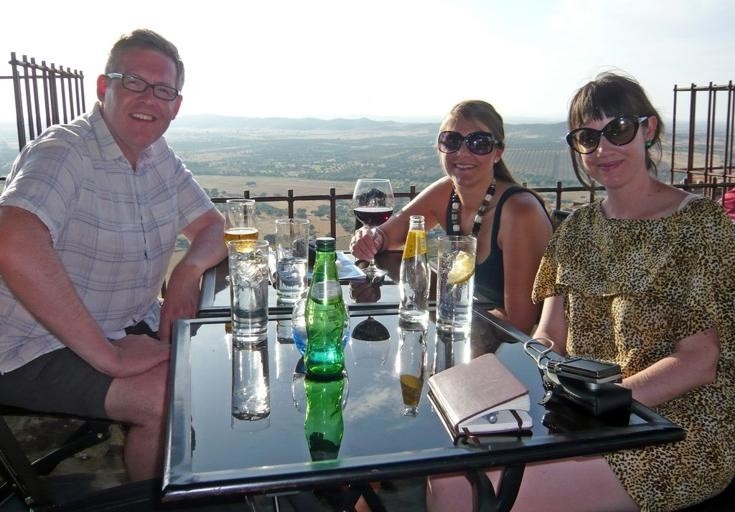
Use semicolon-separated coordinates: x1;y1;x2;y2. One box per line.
0;27;234;481
348;69;734;511
347;102;555;341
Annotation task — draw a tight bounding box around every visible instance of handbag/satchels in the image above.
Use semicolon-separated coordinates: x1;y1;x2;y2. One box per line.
538;363;631;418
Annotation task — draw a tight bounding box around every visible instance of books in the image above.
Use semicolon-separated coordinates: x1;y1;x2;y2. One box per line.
427;352;534;435
426;391;534;446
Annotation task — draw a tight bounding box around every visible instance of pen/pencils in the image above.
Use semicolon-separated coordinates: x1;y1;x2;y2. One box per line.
486;412;498;424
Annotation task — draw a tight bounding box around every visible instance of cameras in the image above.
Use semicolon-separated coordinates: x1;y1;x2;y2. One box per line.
558;357;625;385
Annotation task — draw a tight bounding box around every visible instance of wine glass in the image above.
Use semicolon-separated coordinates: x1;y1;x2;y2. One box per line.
351;176;396;279
349;277;392;369
223;199;258;280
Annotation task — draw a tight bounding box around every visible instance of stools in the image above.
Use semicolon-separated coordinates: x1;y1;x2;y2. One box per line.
0;404;115;511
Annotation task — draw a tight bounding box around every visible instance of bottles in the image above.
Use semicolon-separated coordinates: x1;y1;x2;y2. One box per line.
303;375;345;461
304;235;346;377
398;214;432;333
396;328;426;417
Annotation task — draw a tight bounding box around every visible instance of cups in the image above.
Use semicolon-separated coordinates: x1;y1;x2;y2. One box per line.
230;340;273;434
290;356;350;415
435;234;478;337
276;295;296;346
274;218;310;305
227;238;270;350
292;298;350;359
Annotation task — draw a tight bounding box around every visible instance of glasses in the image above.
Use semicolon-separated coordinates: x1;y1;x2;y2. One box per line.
104;71;181;102
438;130;501;155
566;114;649;155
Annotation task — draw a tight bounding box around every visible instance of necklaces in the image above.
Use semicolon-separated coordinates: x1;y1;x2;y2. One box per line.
451;178;496;248
